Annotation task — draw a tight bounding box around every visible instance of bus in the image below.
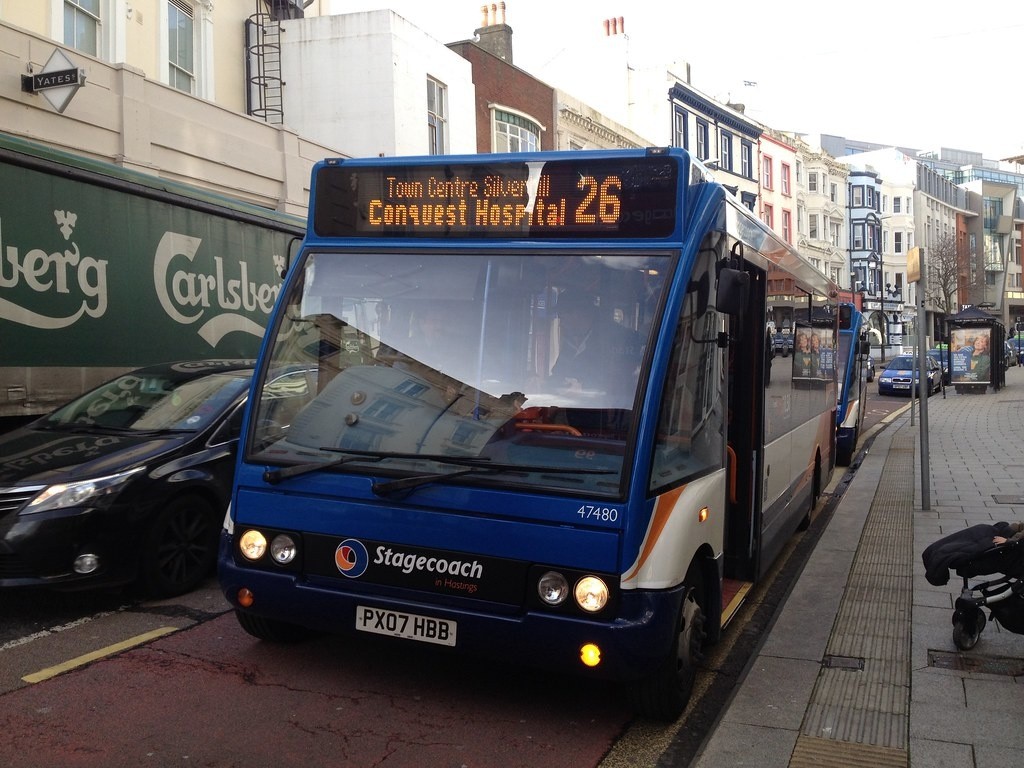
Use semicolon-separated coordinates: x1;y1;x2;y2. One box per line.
217;152;840;717
834;299;873;468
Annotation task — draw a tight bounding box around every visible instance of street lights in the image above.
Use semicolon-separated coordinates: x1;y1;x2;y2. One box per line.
859;212;888;364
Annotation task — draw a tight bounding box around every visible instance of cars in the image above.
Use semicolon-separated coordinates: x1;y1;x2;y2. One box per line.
0;358;333;601
927;348;950;386
1003;336;1024;366
865;355;874;383
878;353;941;397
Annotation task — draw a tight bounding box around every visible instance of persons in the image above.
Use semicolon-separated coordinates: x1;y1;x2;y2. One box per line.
952;334;990;383
550;291;649;403
793;331;832;379
391;302;470;371
765;327;773;388
993;521;1024;545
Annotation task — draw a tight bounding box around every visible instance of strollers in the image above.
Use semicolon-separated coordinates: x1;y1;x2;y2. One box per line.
921;520;1024;652
